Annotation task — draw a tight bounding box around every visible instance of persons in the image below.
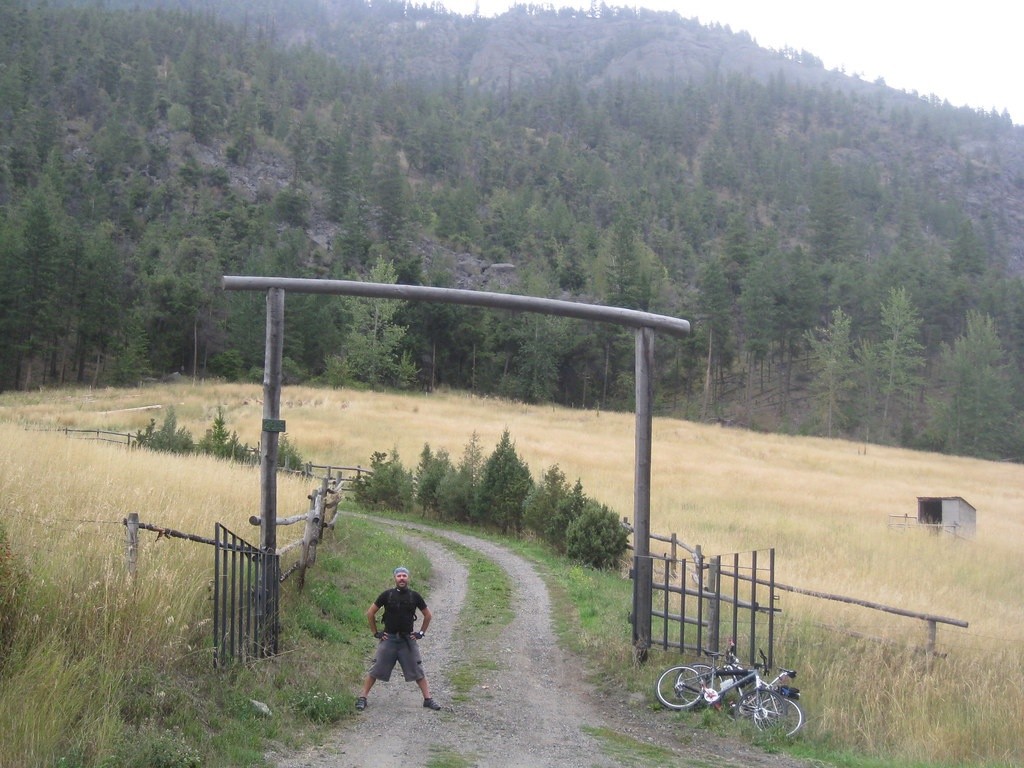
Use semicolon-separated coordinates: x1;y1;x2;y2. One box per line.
355;567;442;710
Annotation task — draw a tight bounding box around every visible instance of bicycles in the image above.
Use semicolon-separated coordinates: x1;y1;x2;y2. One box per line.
655;647;787;732
674;641;805;738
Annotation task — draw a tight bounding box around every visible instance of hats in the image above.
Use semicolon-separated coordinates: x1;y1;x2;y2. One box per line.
394;567;409;576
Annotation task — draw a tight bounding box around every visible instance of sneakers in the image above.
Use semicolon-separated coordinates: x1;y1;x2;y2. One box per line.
423;697;441;710
355;697;367;709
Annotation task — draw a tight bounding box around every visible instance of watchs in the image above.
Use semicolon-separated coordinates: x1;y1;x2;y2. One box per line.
419;631;425;636
